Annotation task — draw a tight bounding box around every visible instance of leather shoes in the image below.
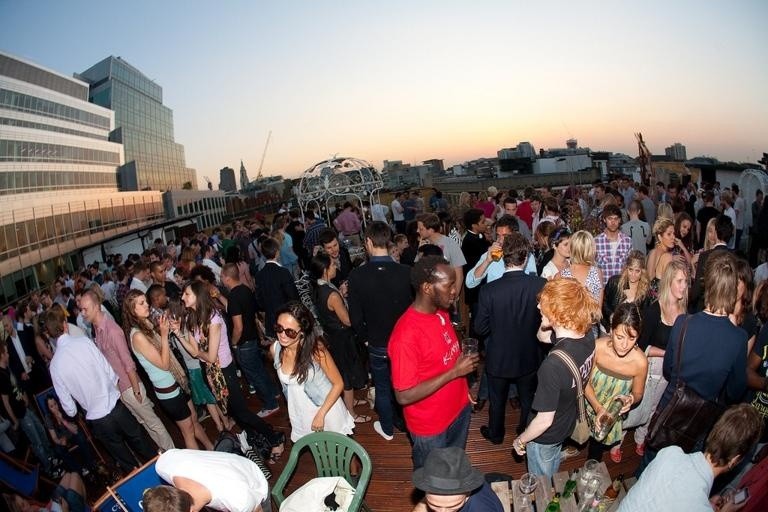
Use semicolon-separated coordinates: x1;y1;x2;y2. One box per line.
509;396;521;410
473;397;486;411
481;425;502;445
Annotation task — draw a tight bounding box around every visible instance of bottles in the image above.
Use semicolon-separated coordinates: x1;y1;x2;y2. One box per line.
516;459;622;512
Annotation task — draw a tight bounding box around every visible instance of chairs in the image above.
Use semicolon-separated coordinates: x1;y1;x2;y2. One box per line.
271;431;373;512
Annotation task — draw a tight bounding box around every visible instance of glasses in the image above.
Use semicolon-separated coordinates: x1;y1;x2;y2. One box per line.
273;322;302;339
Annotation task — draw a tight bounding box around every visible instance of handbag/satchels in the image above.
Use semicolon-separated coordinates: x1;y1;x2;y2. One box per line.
645;385;717;450
212;430;239;452
278;476;355;512
571;418;591;445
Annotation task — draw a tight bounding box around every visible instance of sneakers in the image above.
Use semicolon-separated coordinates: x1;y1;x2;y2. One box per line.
374;421;393;441
561;446;580;462
247;383;280;417
367;387;376;409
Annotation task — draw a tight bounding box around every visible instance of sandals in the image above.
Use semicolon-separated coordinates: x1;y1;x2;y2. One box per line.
353;399;368;407
635;441;645;456
610;445;623;463
269;444;285;465
353;414;372;423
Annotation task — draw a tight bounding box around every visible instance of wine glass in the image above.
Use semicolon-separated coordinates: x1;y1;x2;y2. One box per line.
339;280;349;298
150;306;181;334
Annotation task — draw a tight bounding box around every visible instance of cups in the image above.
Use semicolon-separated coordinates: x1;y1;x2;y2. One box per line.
462;338;479;359
344;239;365;255
491;239;506;262
713;486;738;511
595;400;623;441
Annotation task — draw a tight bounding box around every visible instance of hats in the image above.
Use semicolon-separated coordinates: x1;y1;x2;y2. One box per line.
413;447;485;496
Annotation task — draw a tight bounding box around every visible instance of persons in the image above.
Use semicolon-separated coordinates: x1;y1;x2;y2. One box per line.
1;172;768;512
